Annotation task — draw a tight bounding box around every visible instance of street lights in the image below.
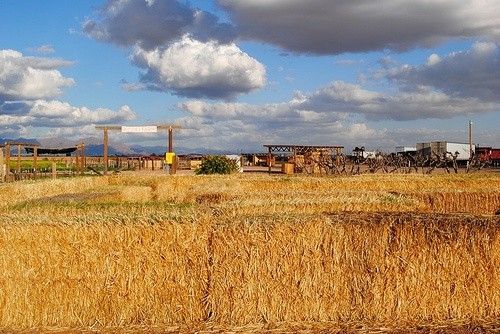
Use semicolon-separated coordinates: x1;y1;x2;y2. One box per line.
82;143;84;176
168;125;172;168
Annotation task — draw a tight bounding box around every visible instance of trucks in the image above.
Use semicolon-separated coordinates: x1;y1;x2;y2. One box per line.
395;146;416;155
415;143;430;151
352;151;383;162
431;141;479;160
475;147;493;168
492;149;500;167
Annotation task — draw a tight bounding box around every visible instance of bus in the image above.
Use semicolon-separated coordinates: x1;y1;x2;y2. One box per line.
189;155;241;170
